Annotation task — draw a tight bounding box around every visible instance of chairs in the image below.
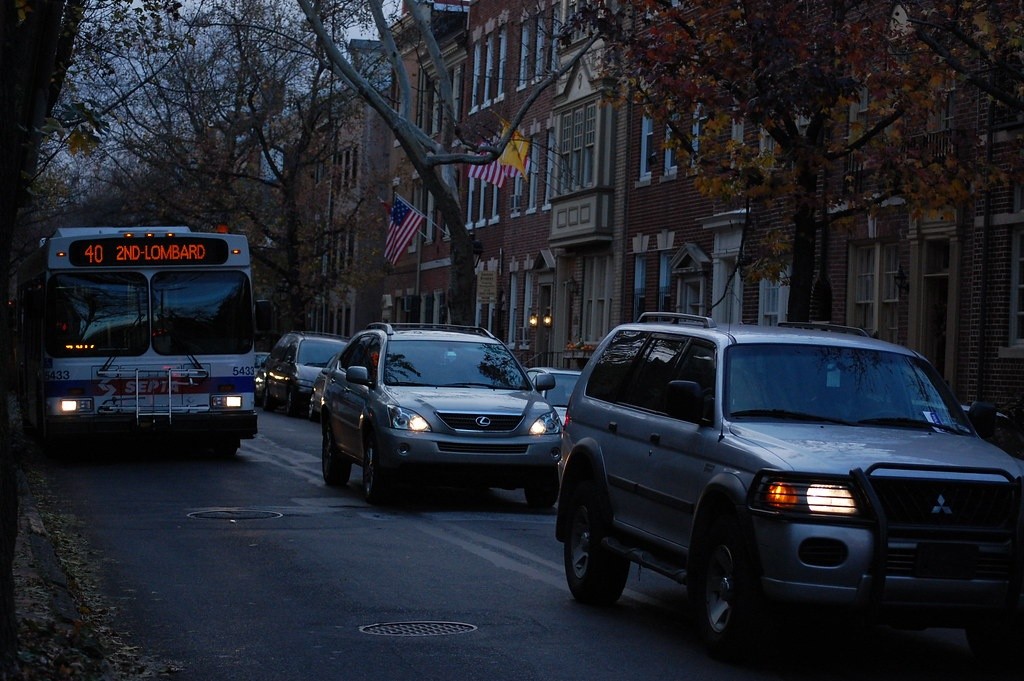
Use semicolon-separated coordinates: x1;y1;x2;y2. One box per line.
829;362;881;420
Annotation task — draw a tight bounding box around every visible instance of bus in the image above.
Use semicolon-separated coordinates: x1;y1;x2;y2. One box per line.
14;224;275;459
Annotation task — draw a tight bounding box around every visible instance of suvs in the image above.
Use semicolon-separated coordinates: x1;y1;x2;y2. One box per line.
320;322;563;510
554;312;1024;667
255;330;351;417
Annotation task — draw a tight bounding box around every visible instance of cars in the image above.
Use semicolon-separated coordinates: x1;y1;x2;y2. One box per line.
524;365;584;428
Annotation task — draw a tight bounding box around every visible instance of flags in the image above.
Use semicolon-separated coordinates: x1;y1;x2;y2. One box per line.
465;121;532;186
384;190;451;265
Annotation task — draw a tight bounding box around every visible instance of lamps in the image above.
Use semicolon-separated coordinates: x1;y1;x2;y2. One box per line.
529;313;539;328
542;313;552;327
